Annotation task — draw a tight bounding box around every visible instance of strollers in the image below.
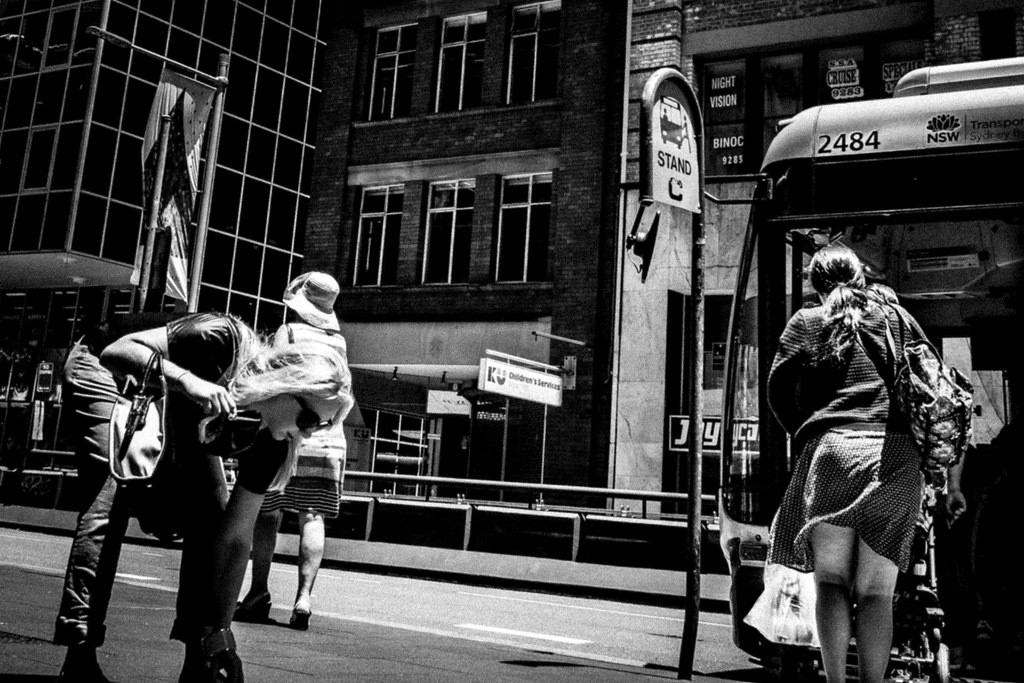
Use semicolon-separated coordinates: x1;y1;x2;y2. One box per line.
783;428;951;683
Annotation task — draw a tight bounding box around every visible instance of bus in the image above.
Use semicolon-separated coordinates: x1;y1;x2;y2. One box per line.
716;55;1023;662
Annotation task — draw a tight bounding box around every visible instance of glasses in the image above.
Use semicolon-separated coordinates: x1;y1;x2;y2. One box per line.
289;394;334;433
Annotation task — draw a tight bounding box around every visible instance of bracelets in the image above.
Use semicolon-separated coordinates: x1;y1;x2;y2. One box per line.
173;370;191;390
201;628;237;656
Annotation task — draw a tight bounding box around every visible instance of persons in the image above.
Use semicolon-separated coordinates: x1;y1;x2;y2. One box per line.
766;243;968;683
232;271;354;631
53;311;355;683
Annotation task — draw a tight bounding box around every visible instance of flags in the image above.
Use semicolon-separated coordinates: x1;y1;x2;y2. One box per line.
130;69;219;304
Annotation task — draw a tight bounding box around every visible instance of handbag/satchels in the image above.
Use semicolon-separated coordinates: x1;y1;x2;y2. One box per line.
109;352;179;498
744;564;819;647
880;303;974;468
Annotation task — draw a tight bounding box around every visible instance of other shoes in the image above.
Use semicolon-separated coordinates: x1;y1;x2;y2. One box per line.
59;644;116;683
178;644;230;683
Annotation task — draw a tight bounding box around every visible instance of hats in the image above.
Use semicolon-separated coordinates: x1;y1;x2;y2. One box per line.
282;271;341;331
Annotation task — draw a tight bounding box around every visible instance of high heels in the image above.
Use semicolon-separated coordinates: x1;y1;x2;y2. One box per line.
234;592;272;622
289;602;311;631
